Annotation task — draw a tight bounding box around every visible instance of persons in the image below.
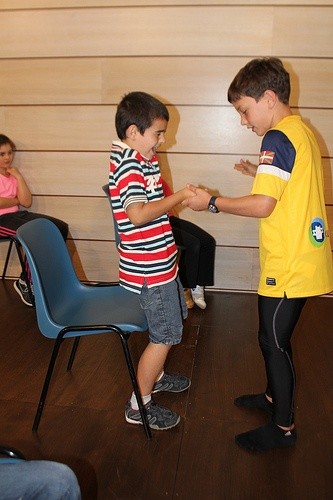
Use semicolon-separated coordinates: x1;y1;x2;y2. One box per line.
162;177;216;309
0;133;69;306
0;461;81;500
181;57;333;453
109;91;207;430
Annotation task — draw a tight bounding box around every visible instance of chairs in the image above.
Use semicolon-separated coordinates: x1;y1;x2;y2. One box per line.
16;218;153;443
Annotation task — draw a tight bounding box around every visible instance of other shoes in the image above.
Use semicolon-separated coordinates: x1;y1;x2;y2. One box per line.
235;421;297;451
191;284;207;309
234;393;274;412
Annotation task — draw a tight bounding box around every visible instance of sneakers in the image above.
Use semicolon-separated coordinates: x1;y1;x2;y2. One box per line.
181;286;195;309
125;399;180;430
152;373;192;394
13;278;35;306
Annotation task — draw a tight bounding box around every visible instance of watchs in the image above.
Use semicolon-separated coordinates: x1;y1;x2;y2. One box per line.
208;195;220;213
